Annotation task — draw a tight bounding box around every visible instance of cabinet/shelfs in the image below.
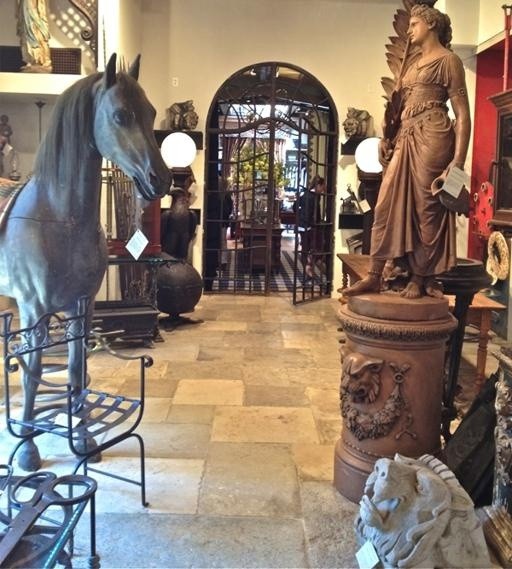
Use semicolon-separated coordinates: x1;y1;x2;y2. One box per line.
477;87;512;340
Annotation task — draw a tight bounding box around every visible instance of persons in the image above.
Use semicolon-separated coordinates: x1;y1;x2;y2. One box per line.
291;175;323;268
218;170;234;269
337;4;473;301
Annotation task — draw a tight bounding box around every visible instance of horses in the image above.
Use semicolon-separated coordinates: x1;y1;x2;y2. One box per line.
0;53;173;472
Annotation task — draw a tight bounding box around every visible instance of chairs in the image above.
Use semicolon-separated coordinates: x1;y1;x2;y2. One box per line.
0;293;153;508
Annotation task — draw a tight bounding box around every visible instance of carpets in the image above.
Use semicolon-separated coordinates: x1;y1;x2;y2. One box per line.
213;246;329;293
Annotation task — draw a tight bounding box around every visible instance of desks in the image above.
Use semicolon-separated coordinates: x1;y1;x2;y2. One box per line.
0;460;104;569
240;218;284;275
339;249;508;375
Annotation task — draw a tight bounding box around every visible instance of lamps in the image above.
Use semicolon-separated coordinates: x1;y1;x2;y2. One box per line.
160;130;197;257
355;135;384;254
